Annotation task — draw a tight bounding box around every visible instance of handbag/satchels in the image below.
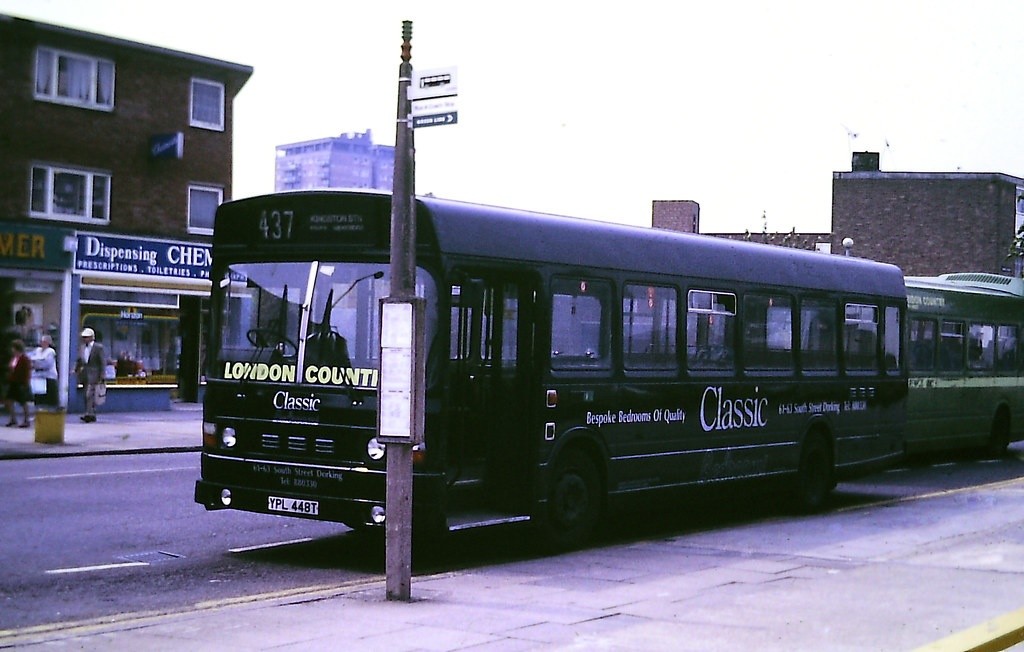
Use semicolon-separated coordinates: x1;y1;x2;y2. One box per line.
96;378;106;405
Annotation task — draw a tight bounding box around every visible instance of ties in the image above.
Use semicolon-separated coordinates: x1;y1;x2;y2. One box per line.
84;343;90;363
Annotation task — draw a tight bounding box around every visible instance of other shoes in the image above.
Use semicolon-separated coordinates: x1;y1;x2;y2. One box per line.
86;415;93;423
6;422;17;426
18;422;30;427
80;416;96;421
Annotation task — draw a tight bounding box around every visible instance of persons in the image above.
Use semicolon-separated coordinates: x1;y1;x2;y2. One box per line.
72;328;105;423
4;339;33;429
25;334;60;408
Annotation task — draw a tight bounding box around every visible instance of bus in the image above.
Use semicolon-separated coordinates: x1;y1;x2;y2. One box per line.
194;189;909;553
809;272;1023;470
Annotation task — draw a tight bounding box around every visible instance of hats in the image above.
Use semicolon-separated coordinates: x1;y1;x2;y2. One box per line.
81;328;94;336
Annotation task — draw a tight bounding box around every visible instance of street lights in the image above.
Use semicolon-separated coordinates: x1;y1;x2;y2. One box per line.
841;236;854;257
